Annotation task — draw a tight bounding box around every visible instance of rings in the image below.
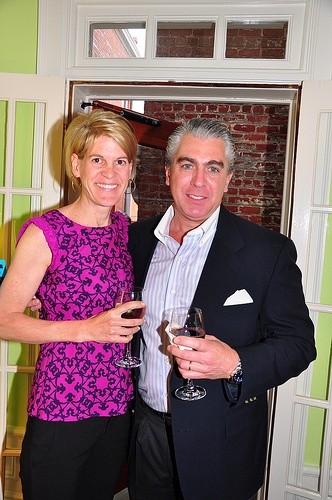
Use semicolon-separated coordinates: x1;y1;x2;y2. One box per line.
188;361;193;371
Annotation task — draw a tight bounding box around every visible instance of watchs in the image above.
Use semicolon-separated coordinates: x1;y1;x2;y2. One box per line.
229;357;243;385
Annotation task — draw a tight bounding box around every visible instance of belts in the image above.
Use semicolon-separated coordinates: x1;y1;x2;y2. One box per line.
141;401;172;426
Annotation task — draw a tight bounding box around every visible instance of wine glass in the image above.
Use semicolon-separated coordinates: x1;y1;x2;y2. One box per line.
168;308;206;400
115;287;146;368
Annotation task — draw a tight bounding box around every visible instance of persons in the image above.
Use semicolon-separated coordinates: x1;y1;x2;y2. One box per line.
27;120;318;500
0;108;145;500
